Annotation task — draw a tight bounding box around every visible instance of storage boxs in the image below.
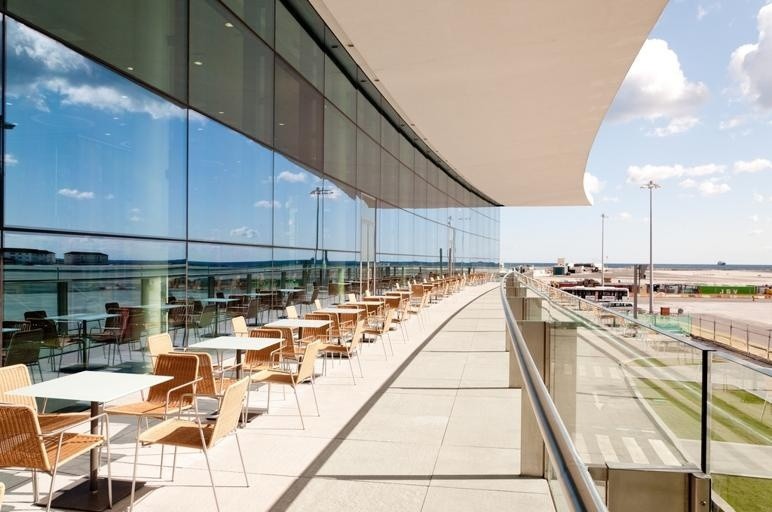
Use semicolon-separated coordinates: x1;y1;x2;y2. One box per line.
661;308;669;315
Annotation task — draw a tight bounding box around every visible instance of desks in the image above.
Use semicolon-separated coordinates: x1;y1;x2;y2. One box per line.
2;371;175;512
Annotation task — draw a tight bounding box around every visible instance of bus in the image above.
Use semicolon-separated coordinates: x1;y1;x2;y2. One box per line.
607;301;635;314
560;285;631;304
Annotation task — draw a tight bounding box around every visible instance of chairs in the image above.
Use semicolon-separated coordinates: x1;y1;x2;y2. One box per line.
0;363;91;503
129;376;250;512
0;402;113;512
99;354;203;478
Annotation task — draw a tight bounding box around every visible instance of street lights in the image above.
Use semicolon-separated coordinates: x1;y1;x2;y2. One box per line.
310;187;332;263
597;213;609;286
447;217;471;279
639;179;662;314
